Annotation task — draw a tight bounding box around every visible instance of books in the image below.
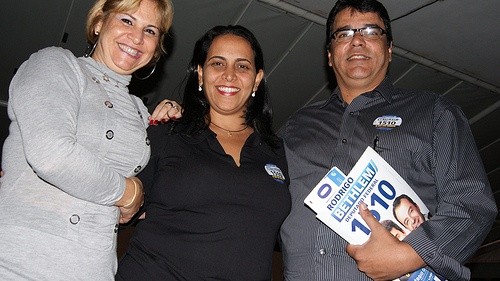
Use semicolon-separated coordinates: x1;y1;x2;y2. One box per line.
315;146;449;281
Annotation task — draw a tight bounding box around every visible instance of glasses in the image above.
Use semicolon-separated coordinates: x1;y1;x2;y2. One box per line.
330;27;386;44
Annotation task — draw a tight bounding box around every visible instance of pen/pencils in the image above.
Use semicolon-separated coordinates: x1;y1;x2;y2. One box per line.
373;135;380;150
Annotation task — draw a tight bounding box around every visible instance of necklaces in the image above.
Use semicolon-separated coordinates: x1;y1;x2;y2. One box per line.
209;121;248;135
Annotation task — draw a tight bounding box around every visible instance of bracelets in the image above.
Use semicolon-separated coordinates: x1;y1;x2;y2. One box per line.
123;178;139;208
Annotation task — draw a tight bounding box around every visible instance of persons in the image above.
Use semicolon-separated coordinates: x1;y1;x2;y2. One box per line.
276;0;498;281
379;219;448;281
0;0;185;281
393;194;432;231
1;25;292;281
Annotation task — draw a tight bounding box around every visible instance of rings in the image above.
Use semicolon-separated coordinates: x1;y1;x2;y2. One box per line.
173;106;179;112
164;102;173;107
